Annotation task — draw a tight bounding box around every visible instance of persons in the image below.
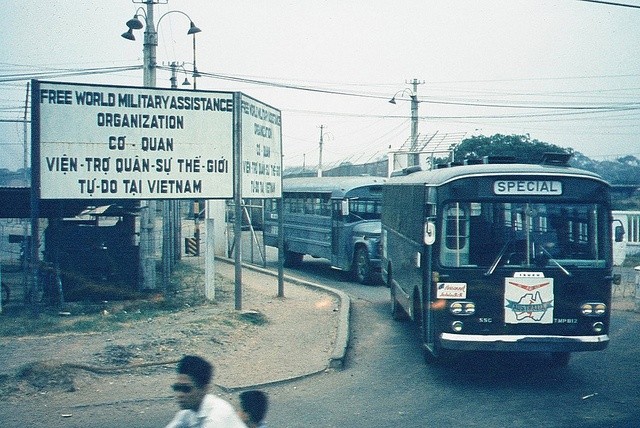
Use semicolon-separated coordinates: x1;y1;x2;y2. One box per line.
236;389;271;428
165;355;246;428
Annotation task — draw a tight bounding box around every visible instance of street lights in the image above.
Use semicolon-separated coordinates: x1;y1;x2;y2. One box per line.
121;7;202;288
165;61;199;272
389;87;421;166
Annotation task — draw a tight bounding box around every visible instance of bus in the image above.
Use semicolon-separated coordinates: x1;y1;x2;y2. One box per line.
262;176;389;285
380;151;625;369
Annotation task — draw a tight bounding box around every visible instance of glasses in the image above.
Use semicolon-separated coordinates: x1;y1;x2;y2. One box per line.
170;384;196;393
